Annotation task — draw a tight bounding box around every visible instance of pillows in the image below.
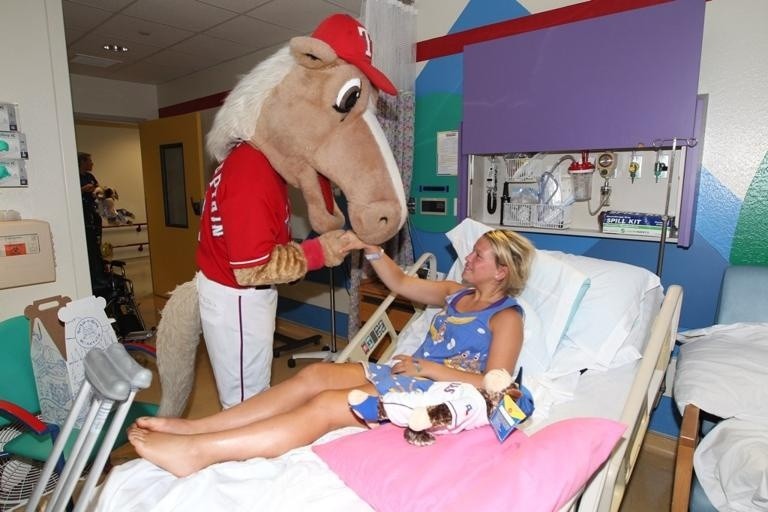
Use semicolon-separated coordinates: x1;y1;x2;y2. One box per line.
445;217;589;394
541;249;660;386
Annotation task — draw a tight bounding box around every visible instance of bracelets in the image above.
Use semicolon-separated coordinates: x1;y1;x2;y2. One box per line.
364;248;384;260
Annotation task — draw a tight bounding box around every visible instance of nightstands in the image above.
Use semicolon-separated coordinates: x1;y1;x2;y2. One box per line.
357;269;415;364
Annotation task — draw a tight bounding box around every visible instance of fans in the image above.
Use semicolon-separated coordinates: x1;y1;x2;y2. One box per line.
1;413;110;512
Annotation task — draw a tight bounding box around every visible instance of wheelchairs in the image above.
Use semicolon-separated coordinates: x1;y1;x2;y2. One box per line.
89;260;157;342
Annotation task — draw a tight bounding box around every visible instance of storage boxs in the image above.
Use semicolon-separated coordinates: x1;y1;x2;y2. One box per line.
24;293;118;430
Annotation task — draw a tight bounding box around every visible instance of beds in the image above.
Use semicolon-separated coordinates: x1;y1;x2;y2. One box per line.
83;217;687;512
669;323;768;511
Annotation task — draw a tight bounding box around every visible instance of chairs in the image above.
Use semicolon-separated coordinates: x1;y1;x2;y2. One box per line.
620;403;701;512
1;316;165;512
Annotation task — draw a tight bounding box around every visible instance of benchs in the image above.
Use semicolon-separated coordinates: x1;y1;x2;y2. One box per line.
276;316;322;357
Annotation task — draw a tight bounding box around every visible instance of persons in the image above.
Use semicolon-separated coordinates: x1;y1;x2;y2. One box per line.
126;227;536;477
77;151;103;275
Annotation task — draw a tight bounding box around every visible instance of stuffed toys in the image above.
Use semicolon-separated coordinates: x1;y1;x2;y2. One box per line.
156;15;409;418
94;186;119;217
